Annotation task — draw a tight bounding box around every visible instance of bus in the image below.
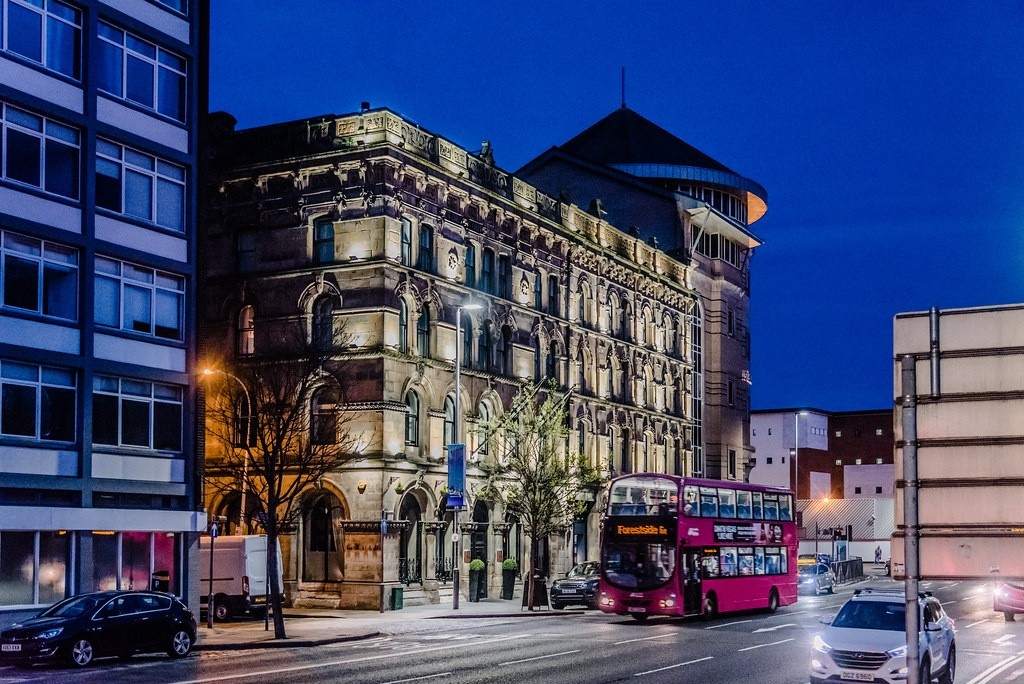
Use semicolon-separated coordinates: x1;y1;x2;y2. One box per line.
600;471;798;622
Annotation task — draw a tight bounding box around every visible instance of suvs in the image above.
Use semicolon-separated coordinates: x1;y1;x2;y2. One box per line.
807;586;955;684
549;560;618;611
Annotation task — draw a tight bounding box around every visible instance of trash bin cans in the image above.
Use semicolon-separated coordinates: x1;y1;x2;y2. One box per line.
520;568;549;611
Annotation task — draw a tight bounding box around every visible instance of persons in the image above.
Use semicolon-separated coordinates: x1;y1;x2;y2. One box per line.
873;546;882;565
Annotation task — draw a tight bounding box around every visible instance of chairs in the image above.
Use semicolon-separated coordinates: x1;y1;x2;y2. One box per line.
725;554;785;575
617;500;791;521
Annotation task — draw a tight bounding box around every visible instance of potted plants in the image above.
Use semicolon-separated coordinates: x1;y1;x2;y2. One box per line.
502;559;518;600
469;559;485;602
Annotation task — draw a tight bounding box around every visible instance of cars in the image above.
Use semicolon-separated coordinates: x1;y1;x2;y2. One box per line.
885;557;891;577
1;589;197;670
993;582;1024;621
797;554;837;571
797;564;837;597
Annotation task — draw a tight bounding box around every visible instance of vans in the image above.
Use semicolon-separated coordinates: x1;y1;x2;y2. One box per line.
196;535;284;622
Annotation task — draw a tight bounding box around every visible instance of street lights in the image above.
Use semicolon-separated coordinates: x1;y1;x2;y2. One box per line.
794;412;807;523
202;366;252;534
452;301;486;610
